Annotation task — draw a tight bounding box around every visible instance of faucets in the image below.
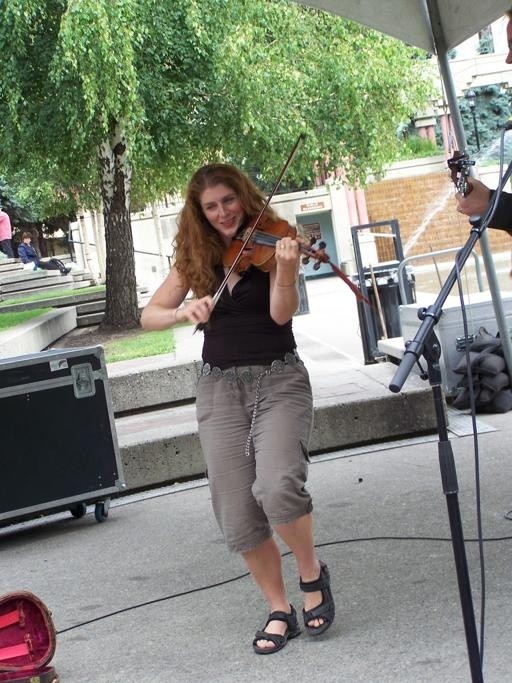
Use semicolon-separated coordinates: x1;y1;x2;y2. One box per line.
253;604;300;654
299;560;334;636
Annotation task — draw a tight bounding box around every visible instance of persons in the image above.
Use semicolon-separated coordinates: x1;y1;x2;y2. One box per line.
0;208;16;264
139;163;336;654
17;231;72;277
454;174;512;236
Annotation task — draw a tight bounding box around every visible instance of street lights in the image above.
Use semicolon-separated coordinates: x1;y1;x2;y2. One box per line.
23;261;35;271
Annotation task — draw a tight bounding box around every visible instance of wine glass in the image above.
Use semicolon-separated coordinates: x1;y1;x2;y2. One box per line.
457;207;464;214
290;243;297;248
279;244;286;249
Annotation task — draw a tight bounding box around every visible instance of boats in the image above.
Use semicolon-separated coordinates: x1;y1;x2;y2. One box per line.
0;345;127;523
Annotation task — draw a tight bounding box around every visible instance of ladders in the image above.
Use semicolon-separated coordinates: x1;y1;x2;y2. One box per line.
223;217;328;272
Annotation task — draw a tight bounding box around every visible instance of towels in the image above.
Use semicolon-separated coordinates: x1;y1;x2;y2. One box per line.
353;259;415;364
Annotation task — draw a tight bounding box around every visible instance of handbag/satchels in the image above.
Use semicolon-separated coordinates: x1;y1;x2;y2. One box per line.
173;307;183;324
272;277;296;291
